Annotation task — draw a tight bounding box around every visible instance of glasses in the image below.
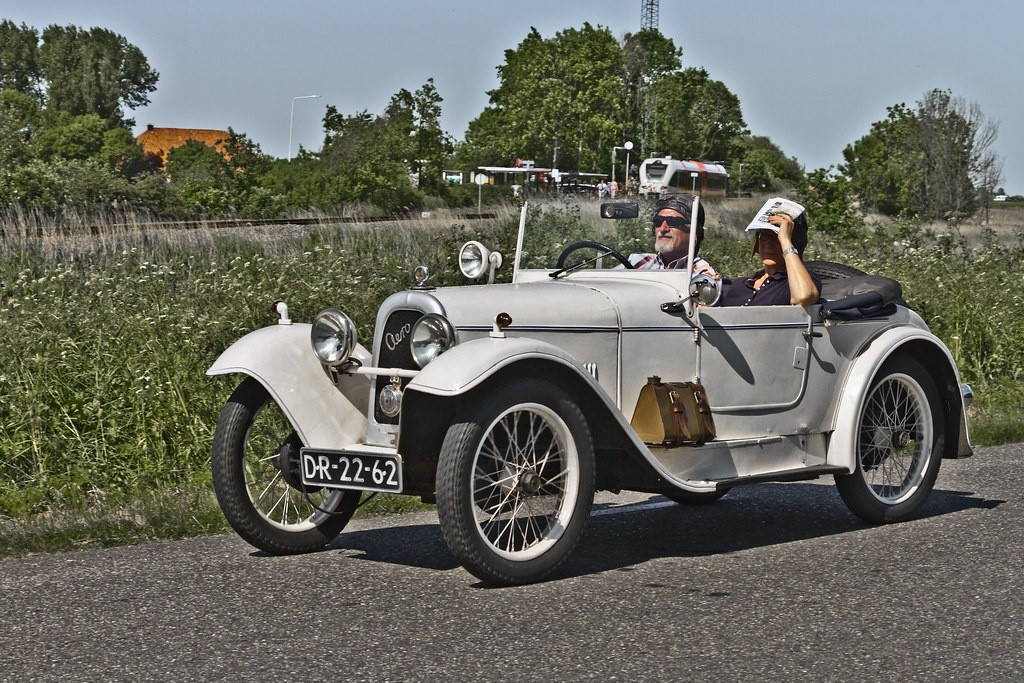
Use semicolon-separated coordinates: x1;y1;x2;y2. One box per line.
650;214;692;228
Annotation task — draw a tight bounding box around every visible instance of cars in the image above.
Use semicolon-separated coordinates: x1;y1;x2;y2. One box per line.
204;192;975;588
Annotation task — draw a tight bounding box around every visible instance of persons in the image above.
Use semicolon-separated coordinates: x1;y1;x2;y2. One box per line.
512;179;522;198
626;176;638;197
597;176;618;199
613;191;719;276
715;209;819;307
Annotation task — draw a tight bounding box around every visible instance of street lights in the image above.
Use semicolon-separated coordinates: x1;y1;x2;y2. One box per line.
611;141;634;197
737;163;750;197
288;94;322;164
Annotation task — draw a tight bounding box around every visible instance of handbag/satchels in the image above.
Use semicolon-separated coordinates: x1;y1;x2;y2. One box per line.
629;375;717;447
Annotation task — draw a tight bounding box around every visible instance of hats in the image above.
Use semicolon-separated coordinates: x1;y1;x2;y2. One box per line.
649;193;706;246
750;209;809;262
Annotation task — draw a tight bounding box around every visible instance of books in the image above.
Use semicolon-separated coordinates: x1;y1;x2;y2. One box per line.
745;197;805;235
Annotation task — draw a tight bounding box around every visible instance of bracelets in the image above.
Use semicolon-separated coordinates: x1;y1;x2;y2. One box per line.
782;246;801;258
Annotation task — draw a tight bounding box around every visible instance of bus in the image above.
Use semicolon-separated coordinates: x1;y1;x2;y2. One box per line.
638;156;728;202
